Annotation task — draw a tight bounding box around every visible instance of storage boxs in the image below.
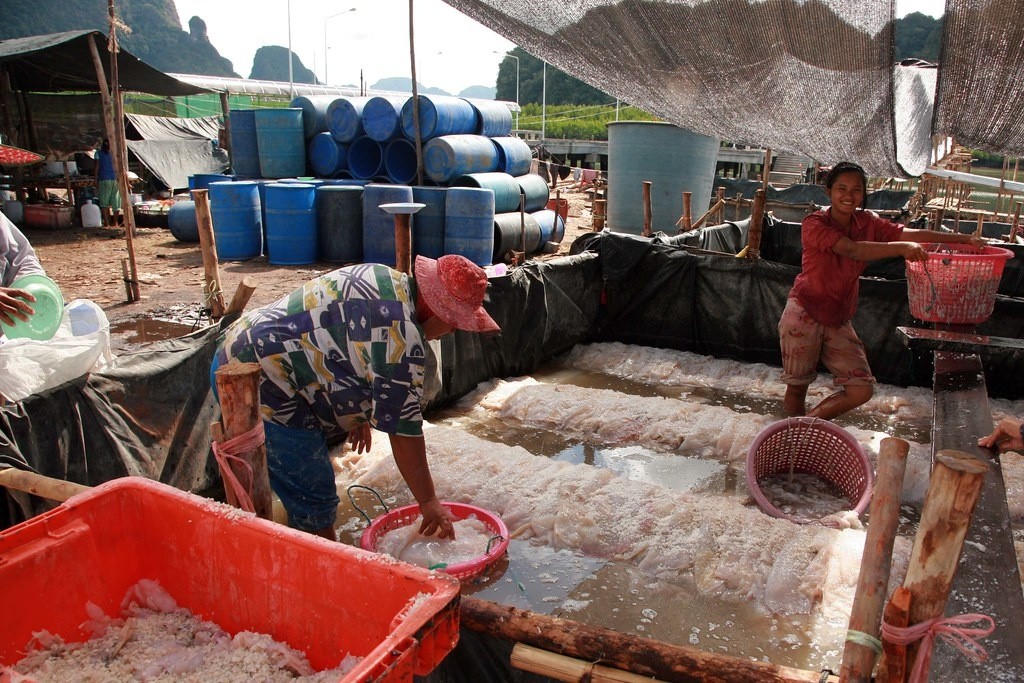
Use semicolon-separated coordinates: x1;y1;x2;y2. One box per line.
0;474;463;683
23;203;72;230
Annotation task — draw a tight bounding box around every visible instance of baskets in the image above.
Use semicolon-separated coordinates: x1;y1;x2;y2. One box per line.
904;242;1016;324
745;416;875;528
347;485;512;579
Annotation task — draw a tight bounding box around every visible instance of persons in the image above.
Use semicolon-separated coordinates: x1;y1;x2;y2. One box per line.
211;254;500;543
94;139;134;229
778;162;988;419
0;209;47;341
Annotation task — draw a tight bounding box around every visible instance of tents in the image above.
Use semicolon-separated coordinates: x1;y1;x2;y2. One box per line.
123;110;230;195
0;30;222;302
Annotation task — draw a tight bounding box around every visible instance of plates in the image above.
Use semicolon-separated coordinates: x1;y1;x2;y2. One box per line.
0;272;65;341
378;203;426;216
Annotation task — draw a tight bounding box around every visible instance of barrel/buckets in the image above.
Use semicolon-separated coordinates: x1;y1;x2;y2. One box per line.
253;109;306;179
166;173;564;268
605;120;719;234
288;94;476;142
80;200;102;227
307;132;499;184
459;97;513;136
227;107;257;179
491;136;534;178
4;201;24;225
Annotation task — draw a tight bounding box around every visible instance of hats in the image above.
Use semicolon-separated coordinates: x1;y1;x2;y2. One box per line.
414;254;502;337
0;144;45;166
